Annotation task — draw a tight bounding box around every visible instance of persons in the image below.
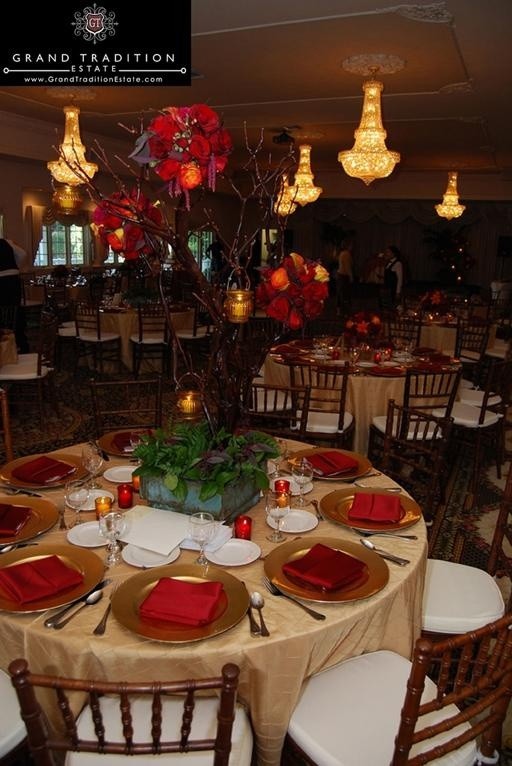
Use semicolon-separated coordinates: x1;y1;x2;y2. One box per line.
367;252;387;303
0;237;30;354
384;246;404;303
118;260;136;270
331;244;360;288
205;235;226;287
245;232;262;275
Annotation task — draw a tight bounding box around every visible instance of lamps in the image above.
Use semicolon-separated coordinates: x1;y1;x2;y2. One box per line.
46;66;465;219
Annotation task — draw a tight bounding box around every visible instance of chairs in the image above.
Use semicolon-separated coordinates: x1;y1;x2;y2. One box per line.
0;262;512;766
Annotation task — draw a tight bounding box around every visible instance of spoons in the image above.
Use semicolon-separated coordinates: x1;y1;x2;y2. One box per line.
54;590;104;631
250;591;270;639
358;537;410;566
342;473;383;484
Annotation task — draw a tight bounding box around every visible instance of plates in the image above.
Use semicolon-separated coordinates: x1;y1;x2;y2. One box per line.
270;333;457;375
263;536;391;604
265;461;320;534
1;495;59;547
99;428;159;458
0;546;107;614
122;537;182;568
1;453;88;490
288;447;373;482
64;440;143;549
111;564;250;644
205;539;263;566
318;486;422;533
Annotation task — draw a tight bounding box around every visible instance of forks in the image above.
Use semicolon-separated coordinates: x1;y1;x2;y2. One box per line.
262;576;327;623
90;580;125;635
352;526;418;541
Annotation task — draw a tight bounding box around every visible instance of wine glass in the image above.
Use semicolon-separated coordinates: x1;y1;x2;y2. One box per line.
186;511;216;562
270;437;287;478
128;430;152;466
264;491;290;544
97;508;127;567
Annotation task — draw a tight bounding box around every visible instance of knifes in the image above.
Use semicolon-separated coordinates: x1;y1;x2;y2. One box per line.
44;578;114;629
240;580;260;638
0;483;43;499
89;439;111;462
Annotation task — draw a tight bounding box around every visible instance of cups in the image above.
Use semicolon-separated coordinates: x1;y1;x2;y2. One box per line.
234;514;252;542
